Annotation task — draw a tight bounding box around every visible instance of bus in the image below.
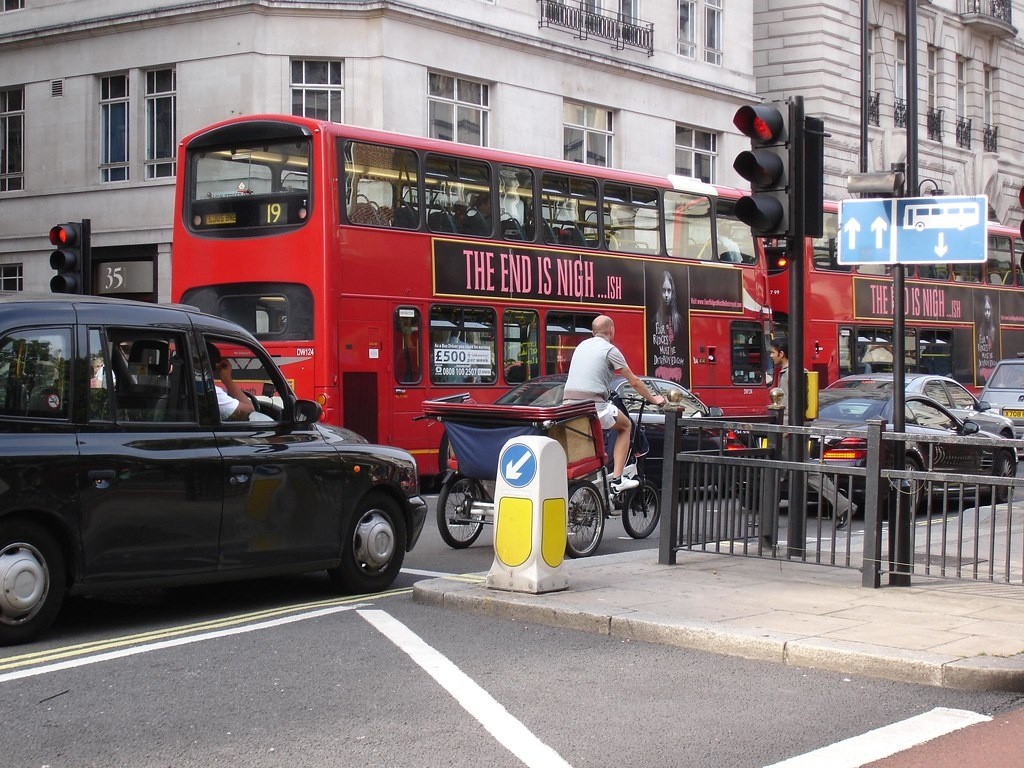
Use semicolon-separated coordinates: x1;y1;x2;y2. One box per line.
672;188;1023;398
172;114;777;480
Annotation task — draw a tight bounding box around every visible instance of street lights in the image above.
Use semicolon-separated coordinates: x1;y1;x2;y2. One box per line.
849;171;911;587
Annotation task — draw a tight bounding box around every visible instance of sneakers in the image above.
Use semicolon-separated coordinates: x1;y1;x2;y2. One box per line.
747;517;760;526
837;504;857;528
610;476;639;494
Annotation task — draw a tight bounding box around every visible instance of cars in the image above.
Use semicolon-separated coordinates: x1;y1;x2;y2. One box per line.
824;374;1017;440
450;375;748;494
722;391;1017;517
0;288;427;646
979;359;1024;439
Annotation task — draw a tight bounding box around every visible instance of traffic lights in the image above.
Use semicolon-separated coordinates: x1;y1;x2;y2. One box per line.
48;220;83;297
764;246;788;274
733;101;791;237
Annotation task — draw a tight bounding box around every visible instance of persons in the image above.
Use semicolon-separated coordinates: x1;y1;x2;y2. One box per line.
470;192;491;226
978;295;995;344
747;337;858;530
717;223;744;264
205;342;255;421
650;270;685;338
562;315;666;495
90;345;138;389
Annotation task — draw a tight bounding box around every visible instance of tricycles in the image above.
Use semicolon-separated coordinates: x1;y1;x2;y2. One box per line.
410;392;663;559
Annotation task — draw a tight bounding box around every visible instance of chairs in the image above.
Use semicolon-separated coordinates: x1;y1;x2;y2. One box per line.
371;200;393;226
428;205;457;235
351;195;381;226
501;210;526;241
529;217;557;244
391;200;419;229
463;207;489;238
558;221;587;247
